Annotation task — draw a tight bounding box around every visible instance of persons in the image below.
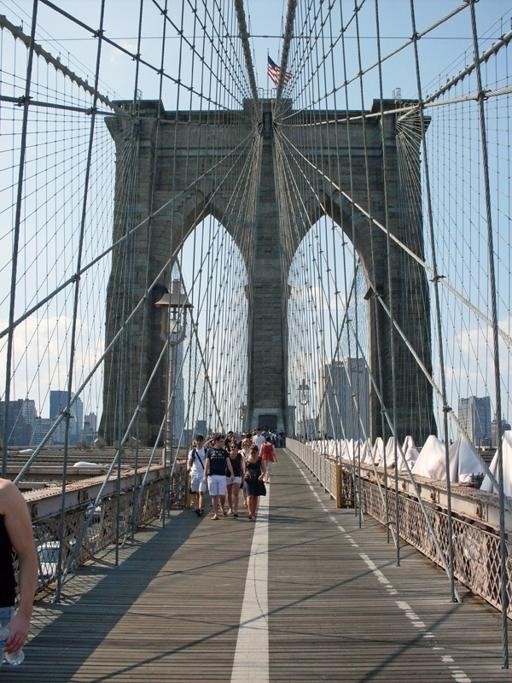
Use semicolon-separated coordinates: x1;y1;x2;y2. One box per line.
184;422;288;523
0;476;40;682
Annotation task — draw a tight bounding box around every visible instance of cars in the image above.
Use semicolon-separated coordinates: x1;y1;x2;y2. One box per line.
33;541;74;592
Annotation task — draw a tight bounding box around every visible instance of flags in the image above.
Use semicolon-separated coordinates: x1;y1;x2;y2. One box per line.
267;55;293;91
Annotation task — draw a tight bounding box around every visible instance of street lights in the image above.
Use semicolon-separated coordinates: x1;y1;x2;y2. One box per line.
153;279;194;466
297;380;311;444
